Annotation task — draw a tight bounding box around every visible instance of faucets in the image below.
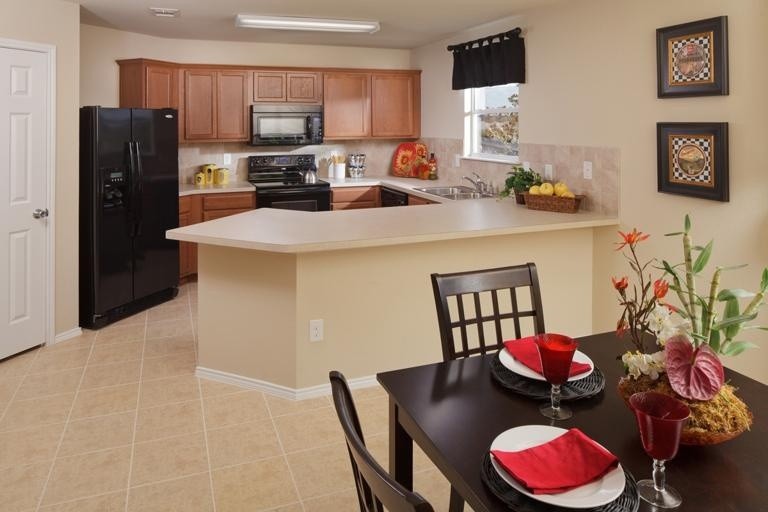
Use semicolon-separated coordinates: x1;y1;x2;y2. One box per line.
462;169;488;194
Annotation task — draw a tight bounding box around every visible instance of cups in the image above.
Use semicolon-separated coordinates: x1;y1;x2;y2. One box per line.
334;162;346;179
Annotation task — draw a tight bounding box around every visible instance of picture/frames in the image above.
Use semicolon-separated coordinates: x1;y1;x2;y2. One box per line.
656;13;732;101
653;119;732;206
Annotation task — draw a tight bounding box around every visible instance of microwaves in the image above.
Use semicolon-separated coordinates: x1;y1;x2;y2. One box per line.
249;104;324;144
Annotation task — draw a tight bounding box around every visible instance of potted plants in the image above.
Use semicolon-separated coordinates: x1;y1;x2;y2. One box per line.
494;162;541;207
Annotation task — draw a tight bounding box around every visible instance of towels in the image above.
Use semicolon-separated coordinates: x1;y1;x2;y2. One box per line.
488;426;621;497
499;331;595;378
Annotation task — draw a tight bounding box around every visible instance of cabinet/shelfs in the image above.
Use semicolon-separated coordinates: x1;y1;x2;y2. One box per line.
178;192;202;286
371;66;423;142
405;194;437;208
115;56;177;109
199;191;256;221
252;64;322;106
320;65;371;142
176;62;253;144
330;184;382;210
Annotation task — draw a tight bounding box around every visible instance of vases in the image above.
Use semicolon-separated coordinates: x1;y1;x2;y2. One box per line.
617;374;751;452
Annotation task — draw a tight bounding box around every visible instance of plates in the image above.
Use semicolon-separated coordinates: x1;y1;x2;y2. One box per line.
498;347;595;382
489;425;626;509
391;141;427;178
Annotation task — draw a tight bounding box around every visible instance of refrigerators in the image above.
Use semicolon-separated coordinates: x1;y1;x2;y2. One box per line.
79;105;179;331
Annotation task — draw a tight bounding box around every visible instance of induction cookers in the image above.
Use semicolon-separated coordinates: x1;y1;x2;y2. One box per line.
251;178;330;192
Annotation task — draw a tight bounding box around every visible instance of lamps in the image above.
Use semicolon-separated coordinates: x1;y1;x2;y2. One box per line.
233;12;383;37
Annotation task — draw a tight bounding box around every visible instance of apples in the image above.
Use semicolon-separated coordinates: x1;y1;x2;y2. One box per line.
529;185;540;195
561;192;575;197
554;182;569;195
540;183;554;196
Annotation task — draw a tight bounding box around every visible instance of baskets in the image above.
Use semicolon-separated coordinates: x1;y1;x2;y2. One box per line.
517;191;585;214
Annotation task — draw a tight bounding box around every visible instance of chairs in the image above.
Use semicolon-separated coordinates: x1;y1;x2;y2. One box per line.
430;261;557;512
322;367;435;512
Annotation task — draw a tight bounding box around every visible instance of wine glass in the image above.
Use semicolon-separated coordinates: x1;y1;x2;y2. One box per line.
629;390;691;510
533;332;579;421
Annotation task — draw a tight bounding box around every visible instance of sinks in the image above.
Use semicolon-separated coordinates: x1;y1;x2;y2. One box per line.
412;185;474;196
441;191;501;201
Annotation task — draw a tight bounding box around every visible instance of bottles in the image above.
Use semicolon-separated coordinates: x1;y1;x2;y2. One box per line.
429;152;438;180
419;155;429;180
346;167;365;178
348;154;366;167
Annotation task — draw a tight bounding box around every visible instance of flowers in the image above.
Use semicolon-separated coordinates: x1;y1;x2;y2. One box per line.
614;210;767;403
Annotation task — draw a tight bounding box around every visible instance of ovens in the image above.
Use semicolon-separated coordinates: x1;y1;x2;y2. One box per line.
256;187;330;211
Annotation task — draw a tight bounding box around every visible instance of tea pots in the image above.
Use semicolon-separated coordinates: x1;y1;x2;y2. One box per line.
298;164;318;184
